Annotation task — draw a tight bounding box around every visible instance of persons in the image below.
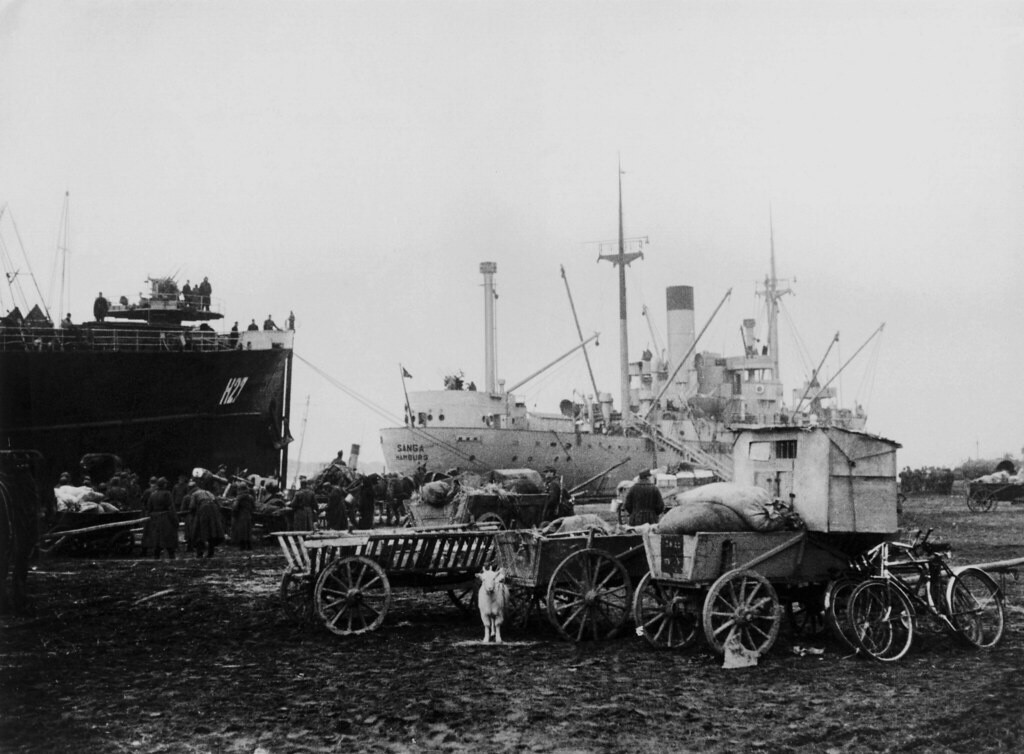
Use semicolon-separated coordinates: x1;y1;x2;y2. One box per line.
542;465;562;522
330;450;347;466
65;313;73;327
379;472;402;525
624;468;665;526
55;469;142;510
230;321;242;350
247;319;259;331
141;475;226;560
263;315;279;330
215;464;256;550
183;276;212;311
93;292;109;322
898;466;956;493
287;474;349;533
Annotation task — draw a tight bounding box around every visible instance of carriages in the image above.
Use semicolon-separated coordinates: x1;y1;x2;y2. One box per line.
309;469;419;526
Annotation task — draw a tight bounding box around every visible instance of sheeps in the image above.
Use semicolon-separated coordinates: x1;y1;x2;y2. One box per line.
476;566;510;645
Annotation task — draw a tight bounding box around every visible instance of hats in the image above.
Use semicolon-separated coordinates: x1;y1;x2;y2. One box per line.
542;465;556;472
638;468;652;477
150;476;157;481
154;476;169;486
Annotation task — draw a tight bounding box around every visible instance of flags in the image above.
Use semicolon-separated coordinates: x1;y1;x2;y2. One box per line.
403;369;412;378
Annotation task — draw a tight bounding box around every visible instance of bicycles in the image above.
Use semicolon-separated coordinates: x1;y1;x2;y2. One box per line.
823;525;1006;662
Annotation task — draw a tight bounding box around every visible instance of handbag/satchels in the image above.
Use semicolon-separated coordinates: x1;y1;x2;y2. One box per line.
545;482;576;516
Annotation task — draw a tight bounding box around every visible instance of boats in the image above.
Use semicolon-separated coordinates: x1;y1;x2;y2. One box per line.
0;186;298;492
373;148;887;497
